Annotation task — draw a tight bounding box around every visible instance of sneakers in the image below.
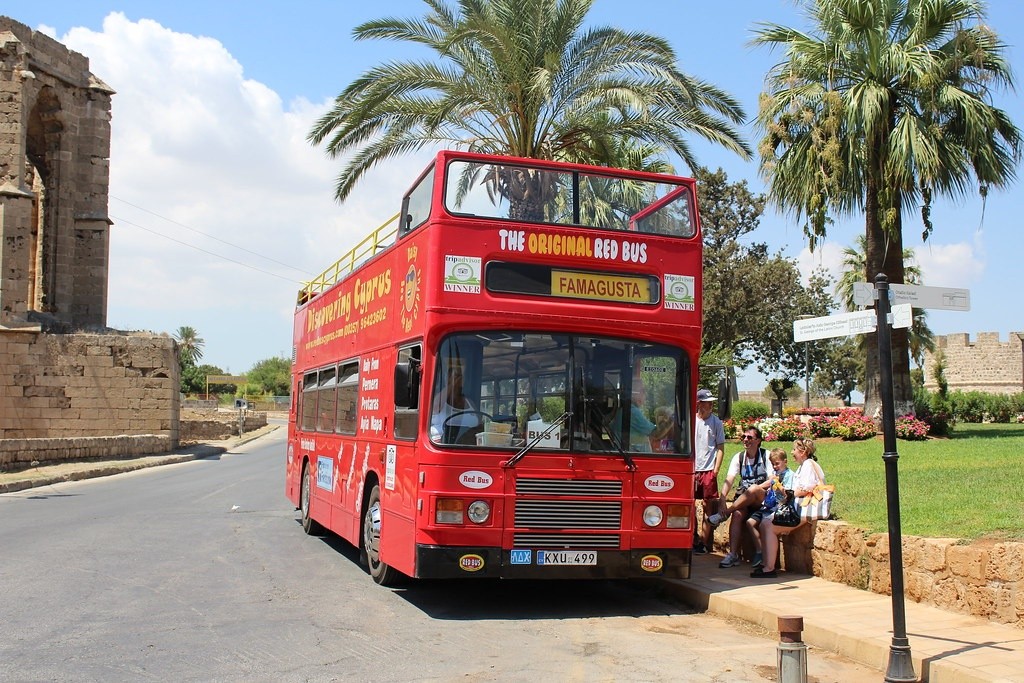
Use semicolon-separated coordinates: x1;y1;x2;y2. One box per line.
706;512;728;525
719;552;741;567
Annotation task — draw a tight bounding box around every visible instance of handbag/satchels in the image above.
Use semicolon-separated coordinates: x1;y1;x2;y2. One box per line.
797;459;833;521
772;490;800;527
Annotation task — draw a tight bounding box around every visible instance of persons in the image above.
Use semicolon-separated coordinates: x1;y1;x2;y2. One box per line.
430;368;482;435
708;427;825;578
610;379;675;453
693;389;724;553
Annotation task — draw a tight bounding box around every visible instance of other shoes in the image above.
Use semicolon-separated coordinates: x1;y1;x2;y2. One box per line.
751;551;763;567
694;542;713;555
750;567;778;579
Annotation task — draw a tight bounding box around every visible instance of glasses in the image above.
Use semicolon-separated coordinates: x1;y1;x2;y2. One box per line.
742;435;759;440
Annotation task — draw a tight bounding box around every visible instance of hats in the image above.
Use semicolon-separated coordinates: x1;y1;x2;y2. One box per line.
697;389;719;402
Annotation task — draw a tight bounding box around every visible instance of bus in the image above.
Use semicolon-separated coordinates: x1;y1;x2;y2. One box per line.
282;149;733;588
